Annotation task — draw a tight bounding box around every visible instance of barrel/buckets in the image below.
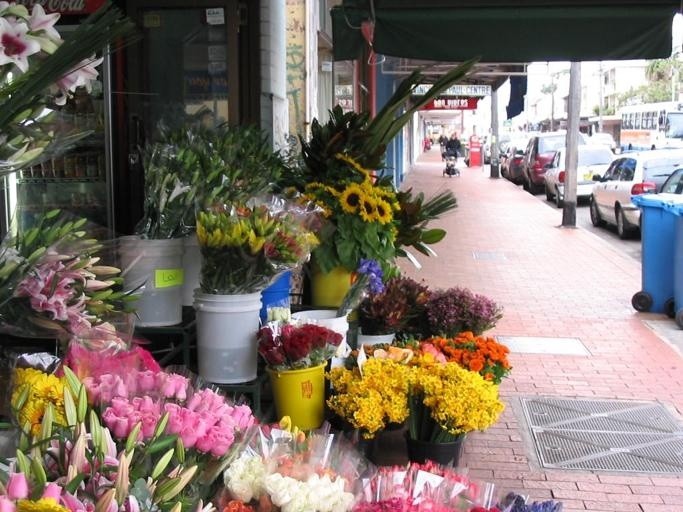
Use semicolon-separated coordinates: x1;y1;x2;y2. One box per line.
292;309;348;361
260;269;294;324
311;257;363;322
190;287;260;384
352;331;396;347
119;235;183;326
263;362;332;438
182;234;205;308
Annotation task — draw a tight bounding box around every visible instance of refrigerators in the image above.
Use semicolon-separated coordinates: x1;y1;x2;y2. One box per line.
0;0;153;295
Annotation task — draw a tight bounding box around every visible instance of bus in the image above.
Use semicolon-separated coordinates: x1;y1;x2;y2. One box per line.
619;101;683;153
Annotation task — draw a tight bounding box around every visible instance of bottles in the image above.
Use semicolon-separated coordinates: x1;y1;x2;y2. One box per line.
14;47;106;209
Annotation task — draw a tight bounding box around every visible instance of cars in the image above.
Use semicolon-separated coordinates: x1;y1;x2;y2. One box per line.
458;127;683;239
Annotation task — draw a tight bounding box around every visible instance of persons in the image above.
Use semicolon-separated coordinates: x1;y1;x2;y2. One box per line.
446;134;460;163
439;133;449;161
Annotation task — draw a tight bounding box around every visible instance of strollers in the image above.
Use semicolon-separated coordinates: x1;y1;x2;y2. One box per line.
442;149;460;178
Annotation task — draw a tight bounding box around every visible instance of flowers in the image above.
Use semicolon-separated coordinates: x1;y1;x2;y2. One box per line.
0;274;571;512
134;105;458;274
1;1;134;273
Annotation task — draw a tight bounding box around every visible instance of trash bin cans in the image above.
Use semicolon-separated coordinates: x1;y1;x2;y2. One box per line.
629;192;683;328
